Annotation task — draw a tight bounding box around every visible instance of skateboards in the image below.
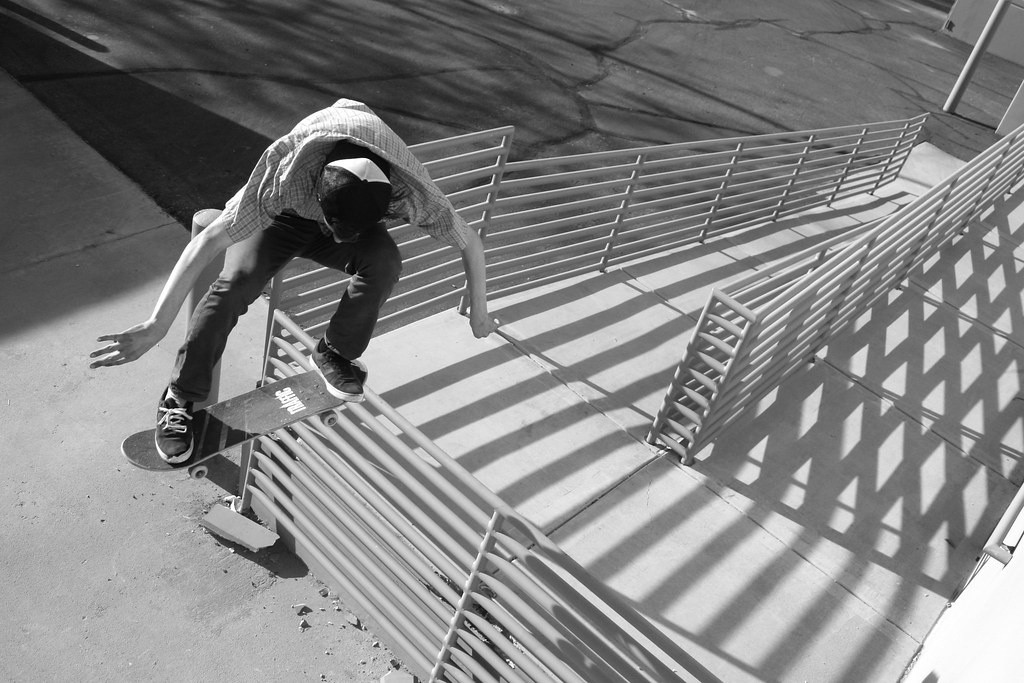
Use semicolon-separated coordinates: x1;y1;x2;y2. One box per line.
120;349;368;480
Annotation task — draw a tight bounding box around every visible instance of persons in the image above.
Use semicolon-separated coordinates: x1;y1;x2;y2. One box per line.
87;97;501;465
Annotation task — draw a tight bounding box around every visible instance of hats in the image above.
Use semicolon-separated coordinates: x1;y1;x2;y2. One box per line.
318;140;391;236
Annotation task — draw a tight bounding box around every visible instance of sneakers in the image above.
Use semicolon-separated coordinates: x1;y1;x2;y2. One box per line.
154;384;194;463
309;337;364;403
